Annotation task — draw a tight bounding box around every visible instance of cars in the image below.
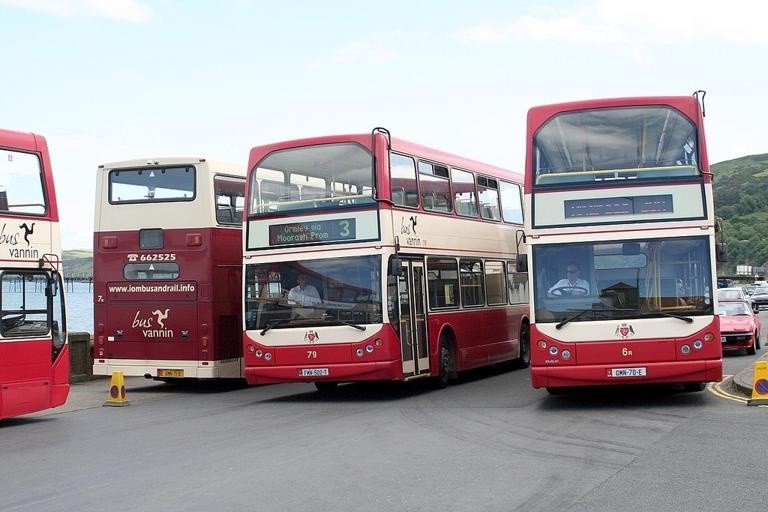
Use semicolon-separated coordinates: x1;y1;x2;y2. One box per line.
718;279;768;355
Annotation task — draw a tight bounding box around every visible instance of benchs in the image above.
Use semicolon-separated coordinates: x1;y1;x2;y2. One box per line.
430;272;503;309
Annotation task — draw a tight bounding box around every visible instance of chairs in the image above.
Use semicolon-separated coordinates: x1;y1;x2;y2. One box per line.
393;186;499;218
217;203;235;224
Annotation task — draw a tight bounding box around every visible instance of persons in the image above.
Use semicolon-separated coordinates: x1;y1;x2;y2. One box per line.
288;272;322;307
547;262;589;298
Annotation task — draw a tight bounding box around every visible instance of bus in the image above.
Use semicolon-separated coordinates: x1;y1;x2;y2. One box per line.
89;157;373;387
0;129;73;420
240;128;532;393
521;90;725;391
